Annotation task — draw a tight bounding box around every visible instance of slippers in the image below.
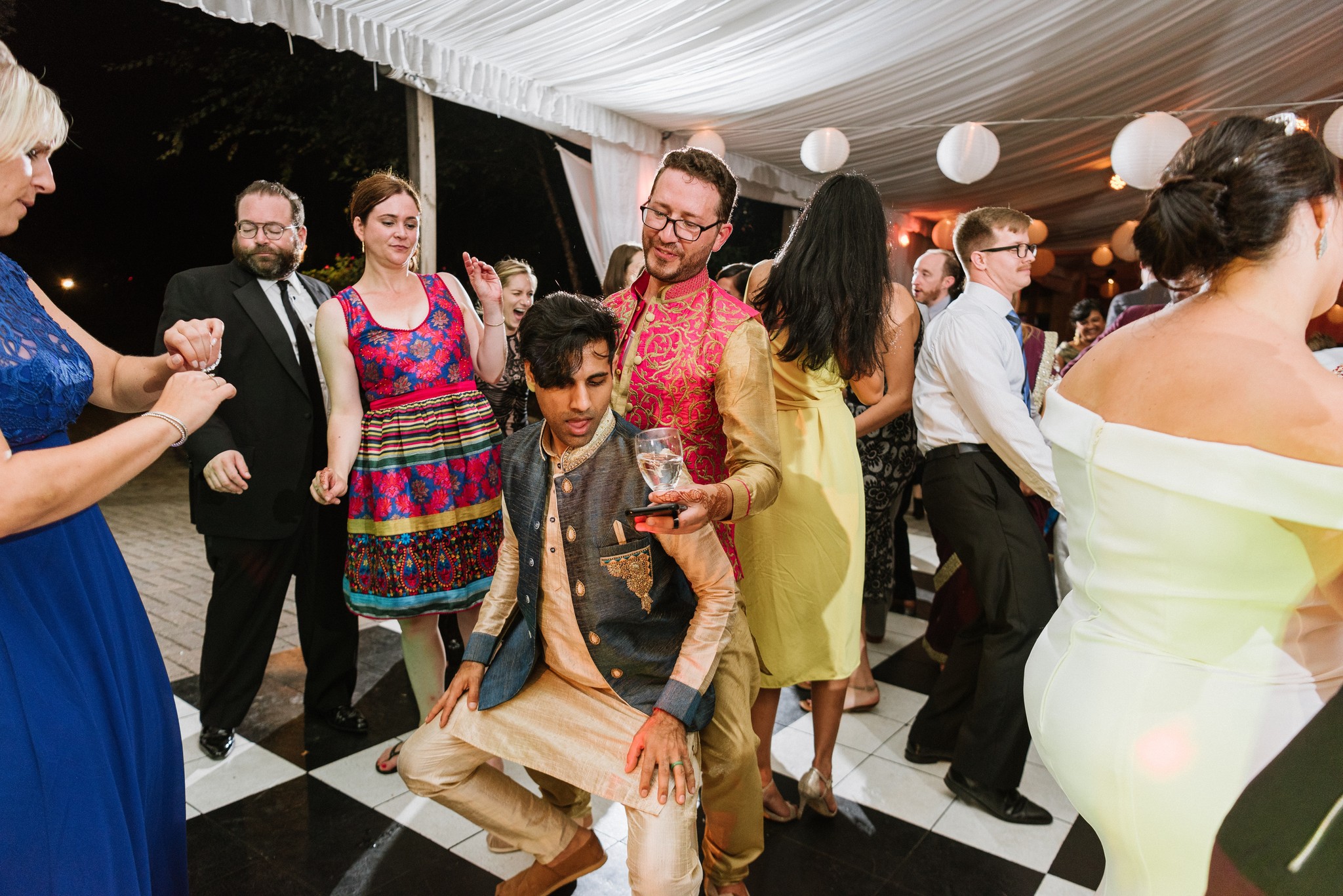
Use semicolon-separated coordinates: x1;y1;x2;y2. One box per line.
375;740;409;774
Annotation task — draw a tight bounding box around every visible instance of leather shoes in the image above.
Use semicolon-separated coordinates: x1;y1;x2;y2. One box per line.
306;707;369;732
905;734;954;764
198;722;234;759
944;764;1053;825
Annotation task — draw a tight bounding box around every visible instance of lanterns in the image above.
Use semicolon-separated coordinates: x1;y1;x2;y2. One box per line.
683;98;1343;190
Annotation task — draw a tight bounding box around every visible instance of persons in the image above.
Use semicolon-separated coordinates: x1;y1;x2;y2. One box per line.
1064;265;1202;376
1024;115;1343;896
157;178;370;760
398;147;897;896
0;43;237;896
439;258;538;697
906;207;1059;823
1054;298;1105;368
309;172;503;777
923;320;1058;670
1105;261;1169;329
798;249;961;712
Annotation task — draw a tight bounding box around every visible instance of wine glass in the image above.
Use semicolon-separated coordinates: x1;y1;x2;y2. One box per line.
635;427;688;510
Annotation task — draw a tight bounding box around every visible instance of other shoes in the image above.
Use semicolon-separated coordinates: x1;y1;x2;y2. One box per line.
487;809;593;851
497;831;607;895
703;874;749;896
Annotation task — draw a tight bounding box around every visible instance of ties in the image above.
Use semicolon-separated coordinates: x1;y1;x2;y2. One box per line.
277;280;327;467
1006;311;1031;416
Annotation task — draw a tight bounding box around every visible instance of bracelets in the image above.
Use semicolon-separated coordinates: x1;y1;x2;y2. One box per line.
482;316;505;326
201;350;222;373
141;411;188;447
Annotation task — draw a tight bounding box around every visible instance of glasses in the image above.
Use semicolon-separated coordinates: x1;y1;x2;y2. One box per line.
640;199;724;242
969;244;1038;262
235;220;301;241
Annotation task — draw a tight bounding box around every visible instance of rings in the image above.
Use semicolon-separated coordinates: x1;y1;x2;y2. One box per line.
314;485;319;491
671;761;683;771
672;514;679;530
208;374;219;389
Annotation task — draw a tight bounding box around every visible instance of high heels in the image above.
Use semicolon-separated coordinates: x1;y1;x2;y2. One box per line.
797;766;837;819
761;780;796;821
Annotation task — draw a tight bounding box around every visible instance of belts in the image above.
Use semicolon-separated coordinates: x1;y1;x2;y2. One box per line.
924;443;992;463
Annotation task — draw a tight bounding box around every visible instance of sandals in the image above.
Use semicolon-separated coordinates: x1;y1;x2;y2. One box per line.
799;683;880;712
797;680;811;691
